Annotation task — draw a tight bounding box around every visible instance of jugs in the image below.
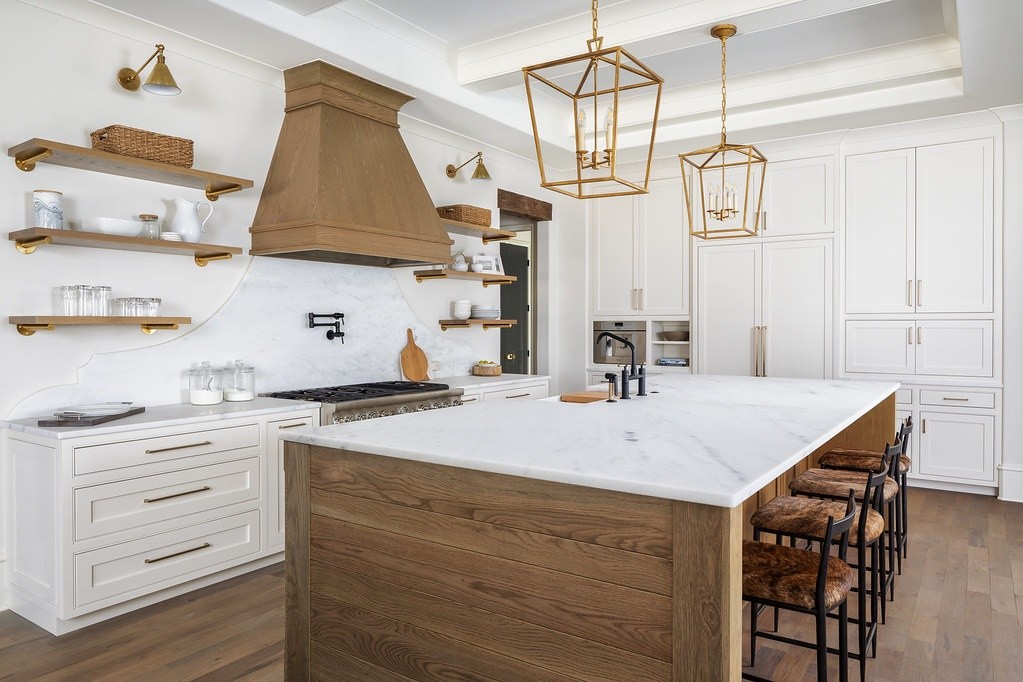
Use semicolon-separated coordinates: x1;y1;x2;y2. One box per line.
171;198;214;243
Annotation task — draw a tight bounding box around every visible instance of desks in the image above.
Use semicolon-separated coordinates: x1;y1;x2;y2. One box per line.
284;372;901;682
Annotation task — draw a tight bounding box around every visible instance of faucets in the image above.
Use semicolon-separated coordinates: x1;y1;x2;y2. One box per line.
596;331;646;398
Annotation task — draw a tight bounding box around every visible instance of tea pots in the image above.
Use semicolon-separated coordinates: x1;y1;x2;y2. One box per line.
471;260;483;273
451;254;469;272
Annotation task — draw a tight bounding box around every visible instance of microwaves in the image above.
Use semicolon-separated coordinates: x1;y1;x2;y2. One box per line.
593;321;646;364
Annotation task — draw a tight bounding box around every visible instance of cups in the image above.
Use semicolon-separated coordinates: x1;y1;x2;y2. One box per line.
52;285;161;317
31;190;63;231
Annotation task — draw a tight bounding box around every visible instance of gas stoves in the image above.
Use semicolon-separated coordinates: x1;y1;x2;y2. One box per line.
258;381;465;426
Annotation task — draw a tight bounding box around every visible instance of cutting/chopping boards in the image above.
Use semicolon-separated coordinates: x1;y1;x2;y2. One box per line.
560;390;633;403
400;329;428;382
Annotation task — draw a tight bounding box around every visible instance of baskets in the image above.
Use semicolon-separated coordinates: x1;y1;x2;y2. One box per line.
435;204;492;228
90;125;194;168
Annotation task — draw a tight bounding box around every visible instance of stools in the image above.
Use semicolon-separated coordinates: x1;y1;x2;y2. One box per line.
741;414;914;682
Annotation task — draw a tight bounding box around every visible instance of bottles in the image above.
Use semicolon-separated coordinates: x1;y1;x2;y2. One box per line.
188;361;224;405
138;213;160;239
224;360;255;402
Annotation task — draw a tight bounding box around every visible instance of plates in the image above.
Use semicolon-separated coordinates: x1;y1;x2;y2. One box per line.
56;404;130;417
479;364;497;367
160;232;182;242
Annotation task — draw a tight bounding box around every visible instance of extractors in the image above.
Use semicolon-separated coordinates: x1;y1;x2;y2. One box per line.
250;61;455;269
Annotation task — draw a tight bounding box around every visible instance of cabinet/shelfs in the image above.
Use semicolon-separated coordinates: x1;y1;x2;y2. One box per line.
420;374;551;406
588;175;691;385
691;156;834;380
8;138;254;335
0;398;322;635
413;218;517;330
839;123;1003;487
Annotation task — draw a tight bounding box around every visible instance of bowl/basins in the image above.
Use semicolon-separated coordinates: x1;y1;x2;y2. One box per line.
472;304;500;319
80;217;143;236
454;299;471;320
657;331;689;341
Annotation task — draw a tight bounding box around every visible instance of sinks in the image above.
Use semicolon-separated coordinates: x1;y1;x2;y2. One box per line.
534;394;605;404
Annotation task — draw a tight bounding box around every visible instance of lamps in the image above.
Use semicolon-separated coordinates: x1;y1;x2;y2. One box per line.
117;44;181;96
522;0;665;199
446;152;492;183
678;24;767;240
